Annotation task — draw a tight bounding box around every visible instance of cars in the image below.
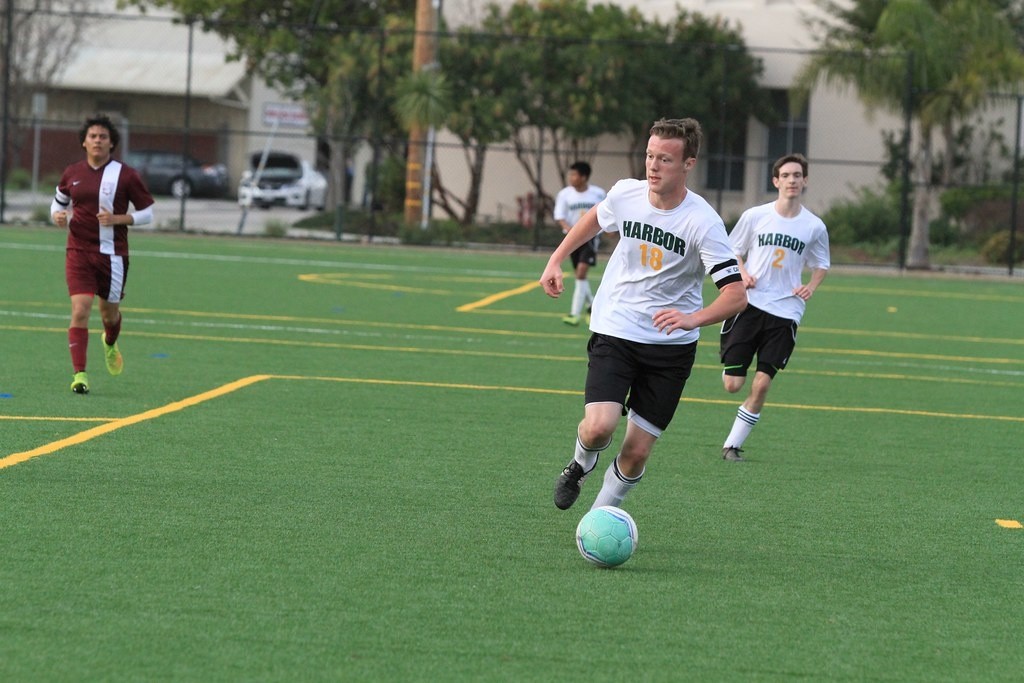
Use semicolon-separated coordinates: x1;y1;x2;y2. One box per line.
121;150;228;199
238;148;328;211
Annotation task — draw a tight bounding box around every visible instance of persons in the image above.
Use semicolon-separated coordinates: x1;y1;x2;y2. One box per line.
719;154;831;460
49;115;156;393
539;118;748;515
554;161;608;325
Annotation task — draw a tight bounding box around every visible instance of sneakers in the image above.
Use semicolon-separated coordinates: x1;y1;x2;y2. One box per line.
554;451;599;510
585;312;591;327
722;448;743;462
563;315;579;326
101;333;123;376
70;371;89;393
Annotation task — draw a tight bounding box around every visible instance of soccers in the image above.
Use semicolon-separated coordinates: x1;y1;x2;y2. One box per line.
575;506;638;568
